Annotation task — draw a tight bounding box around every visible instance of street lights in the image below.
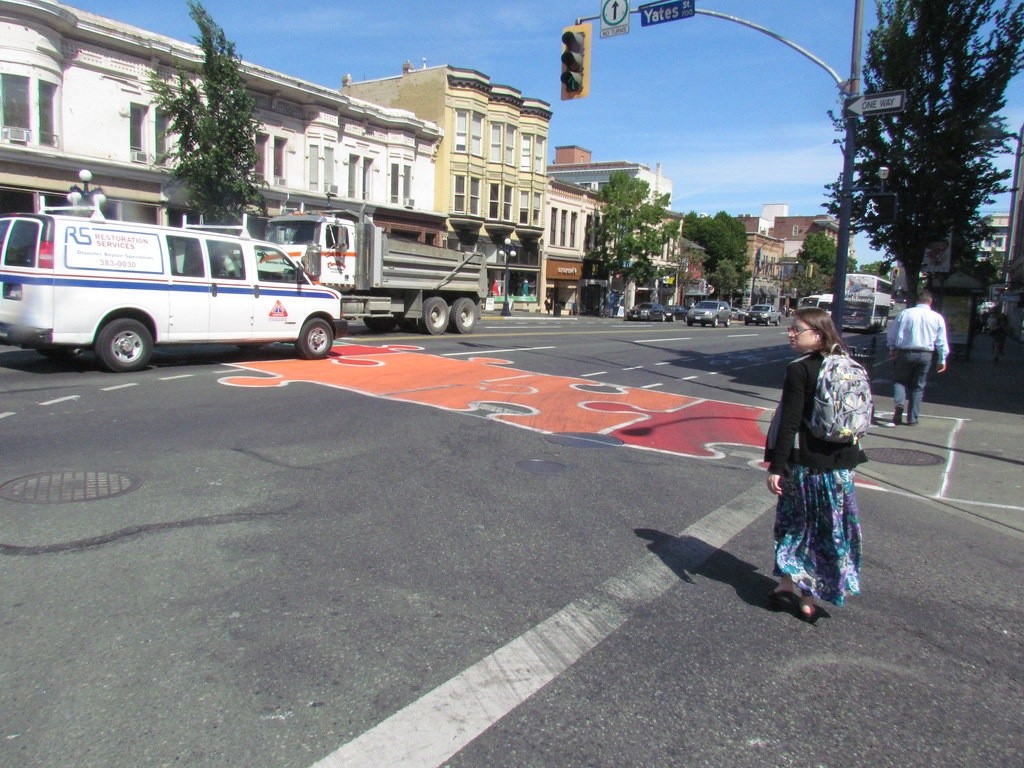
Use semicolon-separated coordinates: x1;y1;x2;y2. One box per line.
500;237;517;316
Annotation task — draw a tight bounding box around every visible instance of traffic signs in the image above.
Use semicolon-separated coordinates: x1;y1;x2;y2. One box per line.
842;89;906;119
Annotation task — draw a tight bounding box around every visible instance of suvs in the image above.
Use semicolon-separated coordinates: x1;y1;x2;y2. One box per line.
687;301;732;328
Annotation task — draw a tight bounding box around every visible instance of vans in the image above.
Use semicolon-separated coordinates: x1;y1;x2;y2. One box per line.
0;205;348;372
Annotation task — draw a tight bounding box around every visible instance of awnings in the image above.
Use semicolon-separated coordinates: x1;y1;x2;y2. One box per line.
753;286;778;298
999;293;1024;302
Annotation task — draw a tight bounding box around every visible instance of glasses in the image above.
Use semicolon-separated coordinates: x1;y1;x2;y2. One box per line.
788;326;817;336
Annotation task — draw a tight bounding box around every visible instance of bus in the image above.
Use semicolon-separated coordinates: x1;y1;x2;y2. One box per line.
842;274;892;330
801;293;833;316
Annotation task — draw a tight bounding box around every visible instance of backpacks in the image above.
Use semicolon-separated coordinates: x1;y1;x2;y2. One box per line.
800;342;874;445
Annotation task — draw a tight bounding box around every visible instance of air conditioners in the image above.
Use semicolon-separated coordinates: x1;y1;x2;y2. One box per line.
130;150;148;164
274;176;286;187
7;127;28;142
403;198;415;208
324;184;338;196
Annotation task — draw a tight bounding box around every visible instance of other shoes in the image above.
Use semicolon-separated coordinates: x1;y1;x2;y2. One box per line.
909;420;918;426
761;588;794;608
797;595;817;623
894;405;904;425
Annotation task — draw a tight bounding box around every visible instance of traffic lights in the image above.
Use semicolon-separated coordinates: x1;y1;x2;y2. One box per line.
561;23;592;101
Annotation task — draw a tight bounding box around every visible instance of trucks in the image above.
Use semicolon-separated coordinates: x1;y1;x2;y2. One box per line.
265;194;488;335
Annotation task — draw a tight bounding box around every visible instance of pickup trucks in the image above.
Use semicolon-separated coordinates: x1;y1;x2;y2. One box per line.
745;304;782;326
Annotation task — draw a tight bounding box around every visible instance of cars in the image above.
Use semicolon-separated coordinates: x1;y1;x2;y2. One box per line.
665;306;688;322
890;299;894;305
627;302;666;321
729;307;746;321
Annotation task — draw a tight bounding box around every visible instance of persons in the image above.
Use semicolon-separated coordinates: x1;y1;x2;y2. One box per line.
976;306;1010;364
523;279;528;296
763;307;868;621
886;290;949;426
545;296;551;314
782;305;787;317
